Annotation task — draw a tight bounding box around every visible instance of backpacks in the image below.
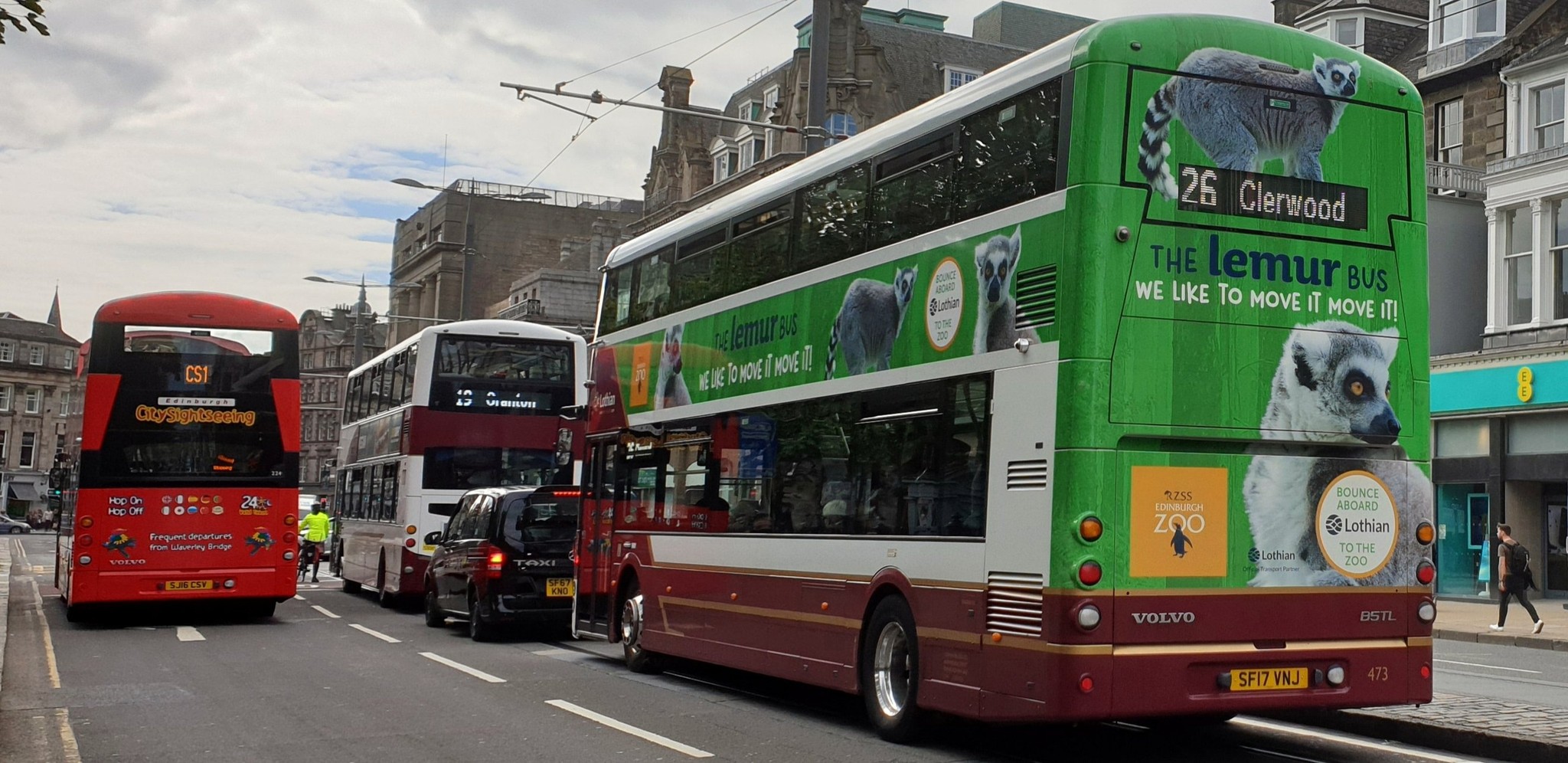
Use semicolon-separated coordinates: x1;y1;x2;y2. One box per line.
1501;541;1539;595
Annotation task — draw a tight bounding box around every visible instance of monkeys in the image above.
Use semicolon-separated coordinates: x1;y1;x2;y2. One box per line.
974;225;1043;353
653;322;694;410
823;263;919;381
1136;44;1360;201
1241;318;1433;588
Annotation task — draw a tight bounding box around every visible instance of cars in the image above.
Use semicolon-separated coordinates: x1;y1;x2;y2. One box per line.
0;515;32;534
300;517;335;561
420;484;613;642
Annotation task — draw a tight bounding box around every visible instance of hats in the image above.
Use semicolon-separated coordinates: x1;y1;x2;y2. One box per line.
1498;522;1510;535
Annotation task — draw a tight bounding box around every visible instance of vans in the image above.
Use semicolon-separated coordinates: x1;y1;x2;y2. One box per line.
297;494;326;522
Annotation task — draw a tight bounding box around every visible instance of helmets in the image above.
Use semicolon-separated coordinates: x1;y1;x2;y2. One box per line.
311;501;321;514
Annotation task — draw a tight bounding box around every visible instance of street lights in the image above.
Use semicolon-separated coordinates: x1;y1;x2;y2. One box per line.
304;275;423;370
393;173;557;321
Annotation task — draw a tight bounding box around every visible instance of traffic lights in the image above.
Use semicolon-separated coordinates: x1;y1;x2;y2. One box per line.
53;488;61;496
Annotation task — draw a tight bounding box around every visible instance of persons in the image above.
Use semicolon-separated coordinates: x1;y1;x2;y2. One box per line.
42;506;54;532
821;499;850;535
298;500;330;583
26;509;43;529
1488;522;1544;634
728;499;772;531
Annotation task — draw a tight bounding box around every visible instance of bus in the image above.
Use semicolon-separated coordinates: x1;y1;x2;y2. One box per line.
550;14;1438;740
320;318;587;606
48;290;302;618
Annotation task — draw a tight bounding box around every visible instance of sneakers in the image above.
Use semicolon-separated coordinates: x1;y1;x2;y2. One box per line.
1531;619;1544;633
1489;621;1504;632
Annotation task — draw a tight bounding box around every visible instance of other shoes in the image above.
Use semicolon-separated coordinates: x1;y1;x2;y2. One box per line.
312;577;319;582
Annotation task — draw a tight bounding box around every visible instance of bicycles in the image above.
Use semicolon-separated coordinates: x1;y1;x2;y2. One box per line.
296;534;319;581
